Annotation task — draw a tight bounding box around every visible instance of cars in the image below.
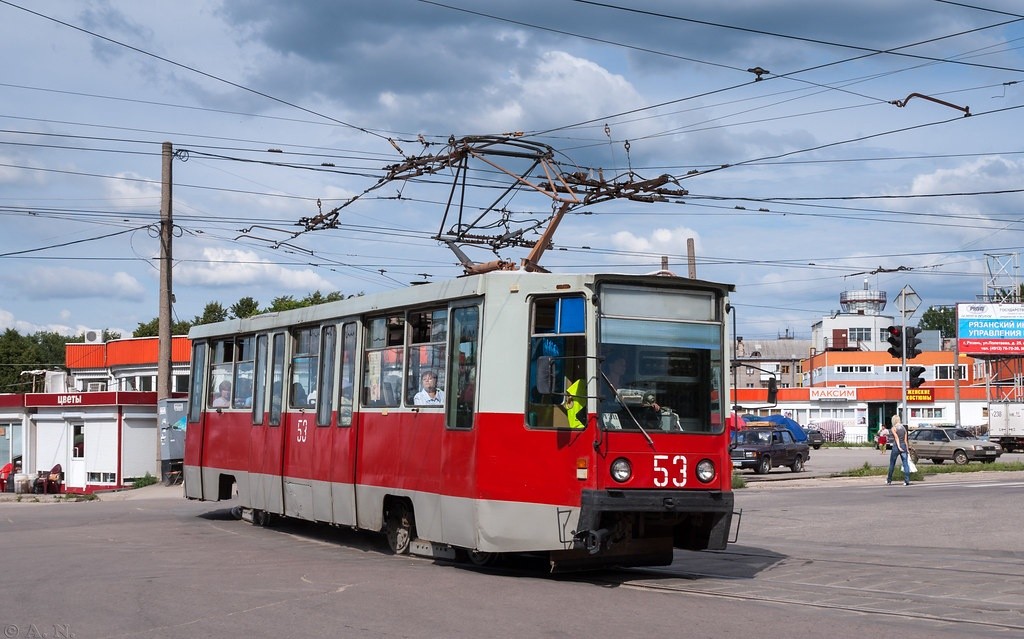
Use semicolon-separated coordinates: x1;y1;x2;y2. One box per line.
907;427;1003;467
729;421;810;476
873;429;895;450
803;428;824;450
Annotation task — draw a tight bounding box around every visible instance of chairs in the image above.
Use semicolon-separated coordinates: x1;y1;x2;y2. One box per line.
31;463;61;495
234;375;418;409
163;462;183;486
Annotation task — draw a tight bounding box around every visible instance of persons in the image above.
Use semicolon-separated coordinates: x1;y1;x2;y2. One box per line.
212;380;231;407
413;371;446;408
601;352;632;412
243;382;308;408
877;425;889;455
342;379;354;406
887;415;909;486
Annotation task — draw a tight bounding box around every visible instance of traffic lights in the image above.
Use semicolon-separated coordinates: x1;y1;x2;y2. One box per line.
906;325;922;359
908;365;926;389
887;324;903;359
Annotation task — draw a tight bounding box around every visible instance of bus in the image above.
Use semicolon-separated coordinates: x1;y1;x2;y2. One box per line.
181;142;779;575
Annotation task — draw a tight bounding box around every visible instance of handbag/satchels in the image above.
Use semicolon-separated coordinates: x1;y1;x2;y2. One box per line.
900;454;917;473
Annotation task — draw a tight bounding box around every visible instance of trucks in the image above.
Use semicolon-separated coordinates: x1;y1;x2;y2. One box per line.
987;402;1024;453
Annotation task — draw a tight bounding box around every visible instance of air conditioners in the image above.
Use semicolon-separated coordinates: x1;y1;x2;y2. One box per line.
85;329;104;343
87;382;106;392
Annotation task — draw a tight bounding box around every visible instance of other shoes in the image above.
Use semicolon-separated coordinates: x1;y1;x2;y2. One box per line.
885;480;891;485
903;483;909;486
